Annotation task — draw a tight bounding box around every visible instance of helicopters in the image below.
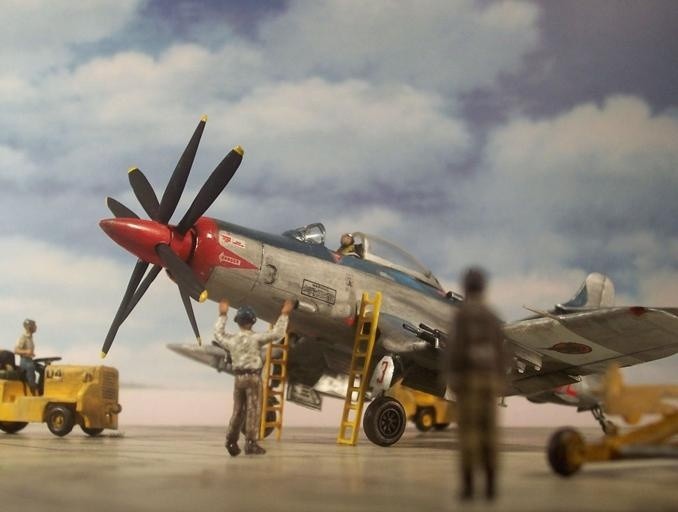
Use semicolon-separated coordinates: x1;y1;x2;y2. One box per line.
97;114;678;478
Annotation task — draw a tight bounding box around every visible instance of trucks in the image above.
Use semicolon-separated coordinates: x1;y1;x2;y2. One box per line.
0;348;121;438
